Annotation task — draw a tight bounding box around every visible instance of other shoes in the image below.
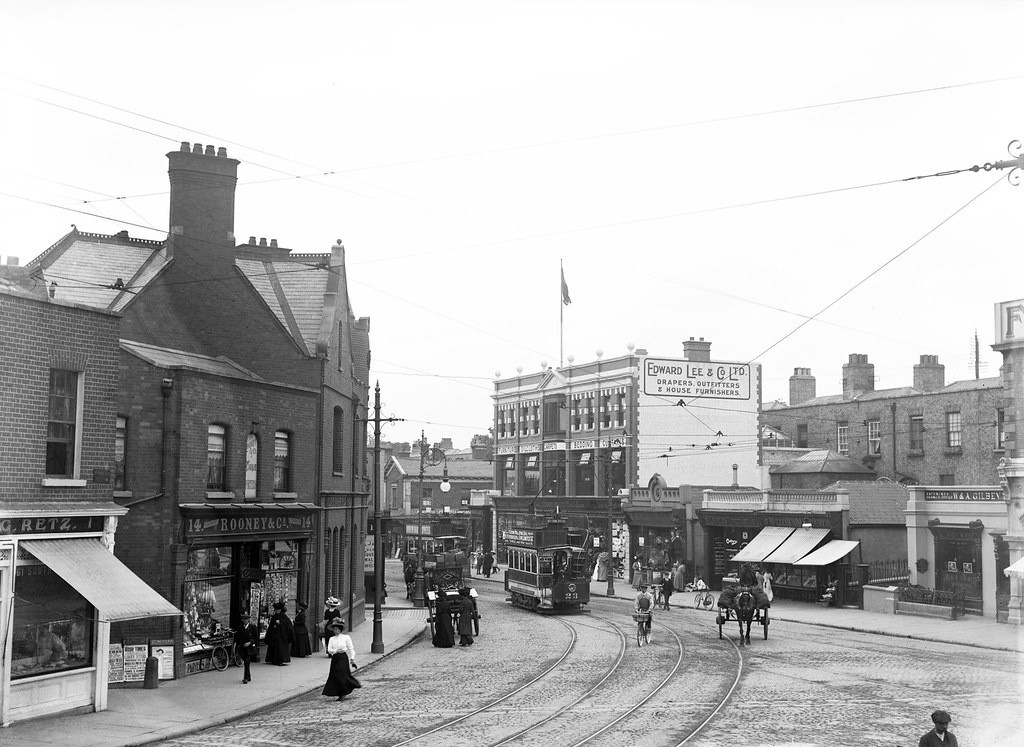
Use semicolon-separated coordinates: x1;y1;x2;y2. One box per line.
335;696;346;701
667;606;670;611
244;678;248;683
242;679;251;681
468;640;474;646
459;644;466;647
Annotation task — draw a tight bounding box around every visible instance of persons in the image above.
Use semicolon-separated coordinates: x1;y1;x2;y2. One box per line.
236;614;257;683
264;603;293;665
324;597;342;657
477;551;500;577
432;592;456;648
739;563;774;602
632;557;643;591
593;549;609;581
634;585;654;634
659;572;674;610
919;710;958;747
674;563;687;591
293;602;312;657
457;591;474;645
322;617;361;700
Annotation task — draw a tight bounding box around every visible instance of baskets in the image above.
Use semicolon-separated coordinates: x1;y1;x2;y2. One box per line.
632;614;648;622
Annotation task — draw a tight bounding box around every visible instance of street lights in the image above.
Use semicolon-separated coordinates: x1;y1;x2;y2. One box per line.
413;447;451;607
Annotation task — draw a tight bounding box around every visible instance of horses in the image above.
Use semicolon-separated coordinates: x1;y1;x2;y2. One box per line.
733;592;756;647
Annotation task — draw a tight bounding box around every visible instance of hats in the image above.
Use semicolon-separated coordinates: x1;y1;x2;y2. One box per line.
665;572;669;575
491;550;497;554
296;602;307;611
326;597;342;606
931;710;951;724
328;617;345;628
272;602;285;610
240;611;251;618
754;566;762;571
640;584;648;587
438;592;447;598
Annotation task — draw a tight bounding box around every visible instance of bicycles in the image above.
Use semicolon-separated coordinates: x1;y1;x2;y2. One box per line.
631;608;651;647
649;584;667;611
694;584;715;611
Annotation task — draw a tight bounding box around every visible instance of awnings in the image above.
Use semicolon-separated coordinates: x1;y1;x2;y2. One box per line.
730;526;859;565
1003;557;1024;579
15;540;182;622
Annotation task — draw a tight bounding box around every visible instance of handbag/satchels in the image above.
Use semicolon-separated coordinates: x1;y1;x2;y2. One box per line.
247;639;258;657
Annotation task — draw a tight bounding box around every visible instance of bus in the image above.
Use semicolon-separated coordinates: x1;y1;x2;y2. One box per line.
403;534;473;582
503;544;593;616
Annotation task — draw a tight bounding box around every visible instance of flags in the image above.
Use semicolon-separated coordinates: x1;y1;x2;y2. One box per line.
563;274;572;305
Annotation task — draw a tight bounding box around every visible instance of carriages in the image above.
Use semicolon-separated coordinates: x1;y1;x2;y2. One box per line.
716;577;771;650
